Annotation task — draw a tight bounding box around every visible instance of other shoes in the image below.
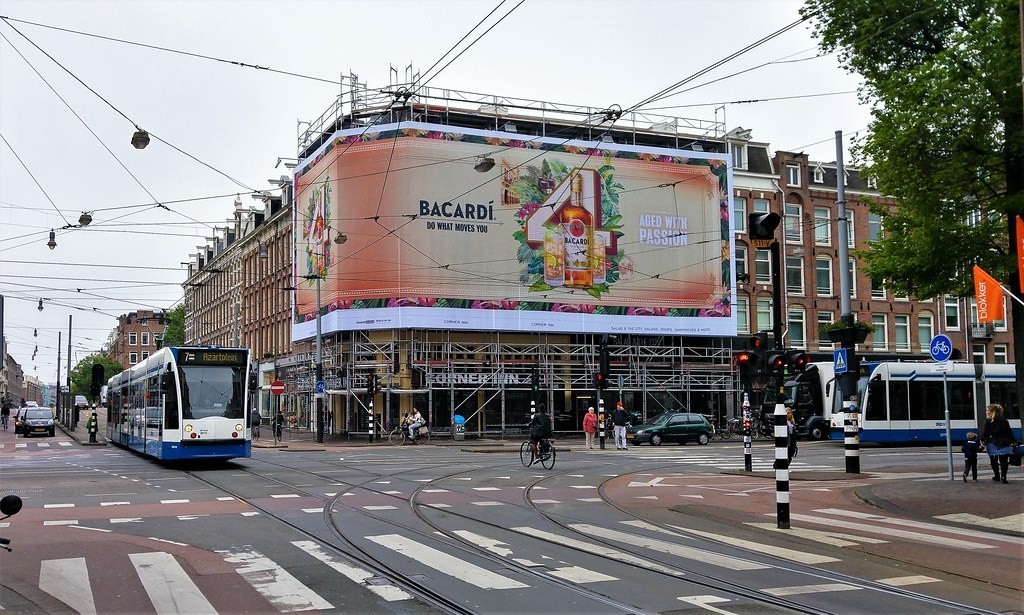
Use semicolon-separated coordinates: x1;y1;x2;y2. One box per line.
533;459;539;464
973;478;979;483
962;473;968;483
616;447;621;450
623;448;628;450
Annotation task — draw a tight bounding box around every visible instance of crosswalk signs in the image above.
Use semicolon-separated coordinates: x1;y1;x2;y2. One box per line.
834;348;848;374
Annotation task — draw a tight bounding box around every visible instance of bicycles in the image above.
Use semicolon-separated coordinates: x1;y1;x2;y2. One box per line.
707;410;766;440
520;424;557;470
604;418;616;443
389;417;430;446
2;416;7;431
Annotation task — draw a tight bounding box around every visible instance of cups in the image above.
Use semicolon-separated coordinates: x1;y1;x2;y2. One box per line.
544;232;565;287
592;234;606;283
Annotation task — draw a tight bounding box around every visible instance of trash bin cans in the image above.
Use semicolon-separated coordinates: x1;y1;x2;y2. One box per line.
453;415;465;440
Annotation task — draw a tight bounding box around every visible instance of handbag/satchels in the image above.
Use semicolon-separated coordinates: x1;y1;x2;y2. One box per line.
1009;444;1022;466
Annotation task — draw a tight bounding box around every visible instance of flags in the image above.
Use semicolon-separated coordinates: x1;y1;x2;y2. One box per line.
974;268;1003;322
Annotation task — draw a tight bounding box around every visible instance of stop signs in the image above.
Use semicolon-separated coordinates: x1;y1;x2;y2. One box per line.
270;381;284;396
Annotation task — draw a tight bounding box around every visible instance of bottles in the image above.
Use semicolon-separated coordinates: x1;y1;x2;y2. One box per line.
560;172;592;289
311;191;325;273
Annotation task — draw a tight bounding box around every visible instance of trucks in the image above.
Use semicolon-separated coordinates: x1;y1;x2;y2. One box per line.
99;385;108;408
761;362;836;441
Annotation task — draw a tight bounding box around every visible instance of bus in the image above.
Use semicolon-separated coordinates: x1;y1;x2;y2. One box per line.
106;344;257;461
825;357;1023;447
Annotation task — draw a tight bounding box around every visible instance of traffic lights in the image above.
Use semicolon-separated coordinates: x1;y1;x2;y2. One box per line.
764;350;786;375
750;331;769;377
749;211;781;240
593;372;604;389
275;368;286;380
367;375;373;394
532;368;540;392
735;350;759;373
375;374;381;394
787;349;806;372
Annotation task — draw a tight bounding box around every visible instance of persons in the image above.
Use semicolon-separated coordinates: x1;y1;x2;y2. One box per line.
399;409;413;438
961;432;984;482
408;407;426;439
529;403;552;464
583;407;598;449
0;404;10;429
271;411;284;436
251;408;262;438
981;404;1016;483
86;414;98;442
612;402;629;451
786;407;797;460
75;405;80;427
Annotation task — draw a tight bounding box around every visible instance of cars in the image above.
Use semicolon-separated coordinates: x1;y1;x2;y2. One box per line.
13;401;58;437
75;395;89;410
626;412;713;446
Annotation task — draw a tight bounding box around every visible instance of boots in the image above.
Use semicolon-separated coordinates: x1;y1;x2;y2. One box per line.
1002;469;1008;484
993;467;1000;481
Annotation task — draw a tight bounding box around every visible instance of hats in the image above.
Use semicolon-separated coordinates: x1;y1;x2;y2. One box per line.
617;402;623;405
